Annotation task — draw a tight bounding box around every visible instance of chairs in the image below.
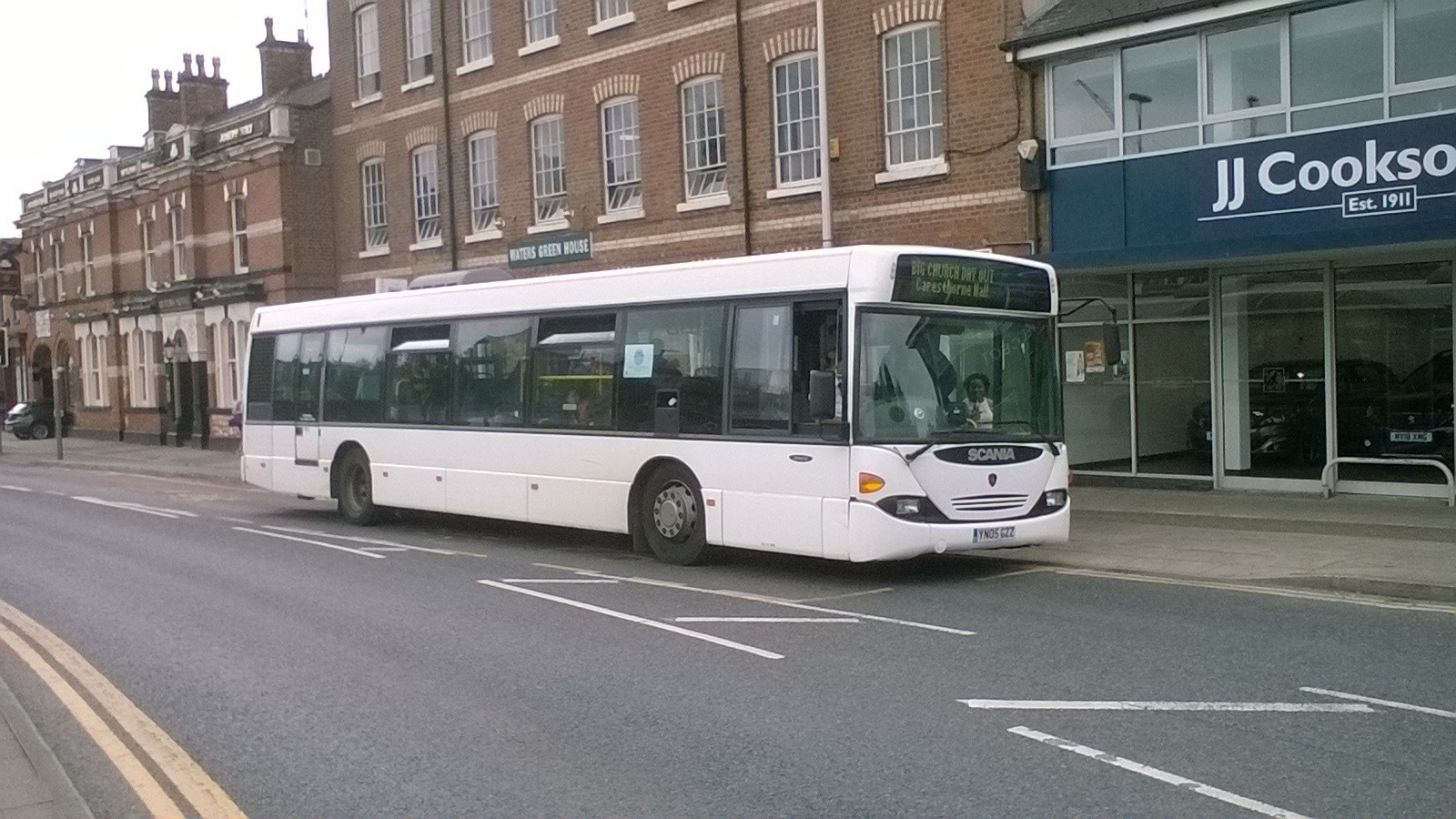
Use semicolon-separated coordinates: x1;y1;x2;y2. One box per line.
274;362;726;430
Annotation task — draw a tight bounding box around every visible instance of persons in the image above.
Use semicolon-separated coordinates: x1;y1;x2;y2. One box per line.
963;374;995;429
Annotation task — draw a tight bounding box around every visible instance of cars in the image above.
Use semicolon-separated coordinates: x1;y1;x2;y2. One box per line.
1357;347;1455;476
1183;358;1401;470
3;397;68;441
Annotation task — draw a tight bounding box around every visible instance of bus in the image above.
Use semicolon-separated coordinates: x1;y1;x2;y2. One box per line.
237;242;1123;569
